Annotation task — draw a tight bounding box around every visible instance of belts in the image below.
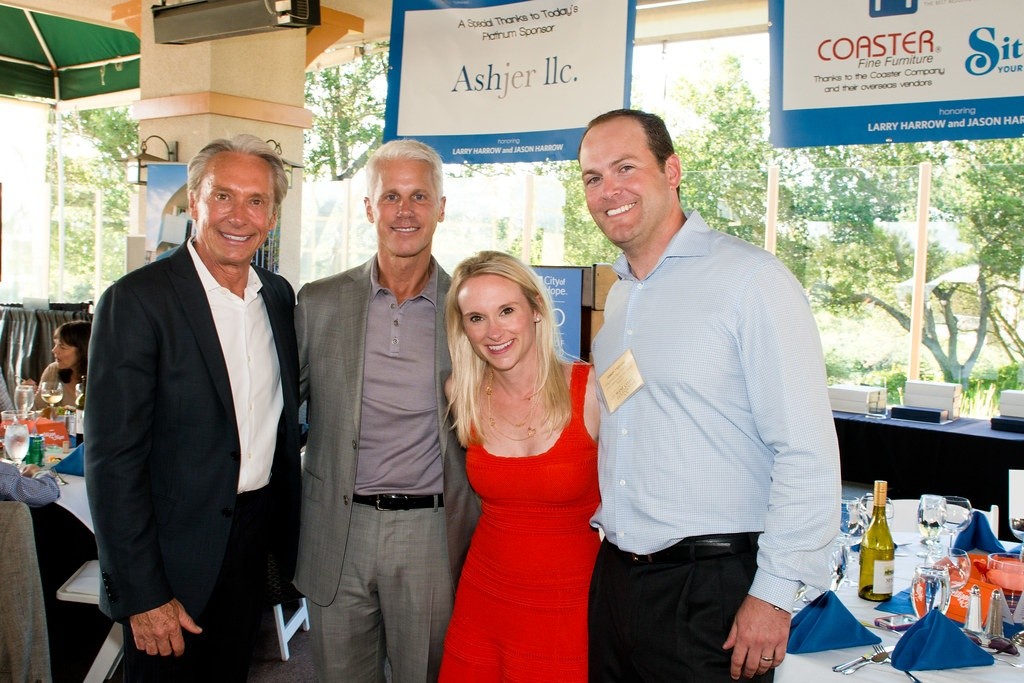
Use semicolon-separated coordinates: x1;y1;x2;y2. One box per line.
352;493;444;511
632;536;750;565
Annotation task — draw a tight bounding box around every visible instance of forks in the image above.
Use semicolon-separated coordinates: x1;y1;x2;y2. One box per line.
872;643;923;683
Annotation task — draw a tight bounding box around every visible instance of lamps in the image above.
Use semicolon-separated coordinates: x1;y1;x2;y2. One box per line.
268;139;304;189
118;136;179;188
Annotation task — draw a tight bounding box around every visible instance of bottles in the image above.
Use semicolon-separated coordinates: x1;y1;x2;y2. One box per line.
962;584;986;633
857;479;895;602
74;375;88;447
983;589;1006;639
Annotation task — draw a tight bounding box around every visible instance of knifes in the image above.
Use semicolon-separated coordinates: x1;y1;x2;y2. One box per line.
833;644;895;672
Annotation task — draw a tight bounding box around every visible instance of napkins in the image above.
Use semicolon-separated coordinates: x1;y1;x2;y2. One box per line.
51;441;83;477
892;609;994;670
787;590;881;654
954;513;1006;554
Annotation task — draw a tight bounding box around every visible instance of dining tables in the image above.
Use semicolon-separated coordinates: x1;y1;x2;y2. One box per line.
774;533;1024;683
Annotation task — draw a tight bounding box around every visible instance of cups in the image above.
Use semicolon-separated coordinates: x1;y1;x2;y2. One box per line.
44;446;63;469
4;424;30;465
0;410;36;436
909;564;952;621
866;386;889;419
915;493;947;545
925;547;972;591
13;384;36;413
840;493;894;544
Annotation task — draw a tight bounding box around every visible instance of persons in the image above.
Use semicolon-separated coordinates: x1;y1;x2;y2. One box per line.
0;460;60;507
438;251;593;683
0;367;14;412
294;140;476;683
586;108;843;683
24;321;91;410
85;136;303;683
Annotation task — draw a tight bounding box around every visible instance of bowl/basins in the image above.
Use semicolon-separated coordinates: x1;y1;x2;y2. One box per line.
986;552;1024;592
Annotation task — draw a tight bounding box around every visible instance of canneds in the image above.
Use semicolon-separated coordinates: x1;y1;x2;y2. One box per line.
25;434;44;467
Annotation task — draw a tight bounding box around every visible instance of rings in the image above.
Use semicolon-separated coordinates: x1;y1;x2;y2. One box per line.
762;657;774;661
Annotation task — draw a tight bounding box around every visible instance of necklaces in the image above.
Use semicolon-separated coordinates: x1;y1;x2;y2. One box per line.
485;370;547;441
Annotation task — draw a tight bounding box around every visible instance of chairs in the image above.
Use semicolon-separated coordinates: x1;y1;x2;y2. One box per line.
57;560;125;683
867;493;1000;540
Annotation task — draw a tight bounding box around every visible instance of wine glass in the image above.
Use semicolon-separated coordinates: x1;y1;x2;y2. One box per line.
40;381;64;420
941;496;974;547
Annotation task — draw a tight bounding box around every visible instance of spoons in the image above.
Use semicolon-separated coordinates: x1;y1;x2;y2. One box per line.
841;651;888;675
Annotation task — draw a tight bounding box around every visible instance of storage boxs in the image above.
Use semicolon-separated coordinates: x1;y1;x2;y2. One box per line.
828;381;1024;418
